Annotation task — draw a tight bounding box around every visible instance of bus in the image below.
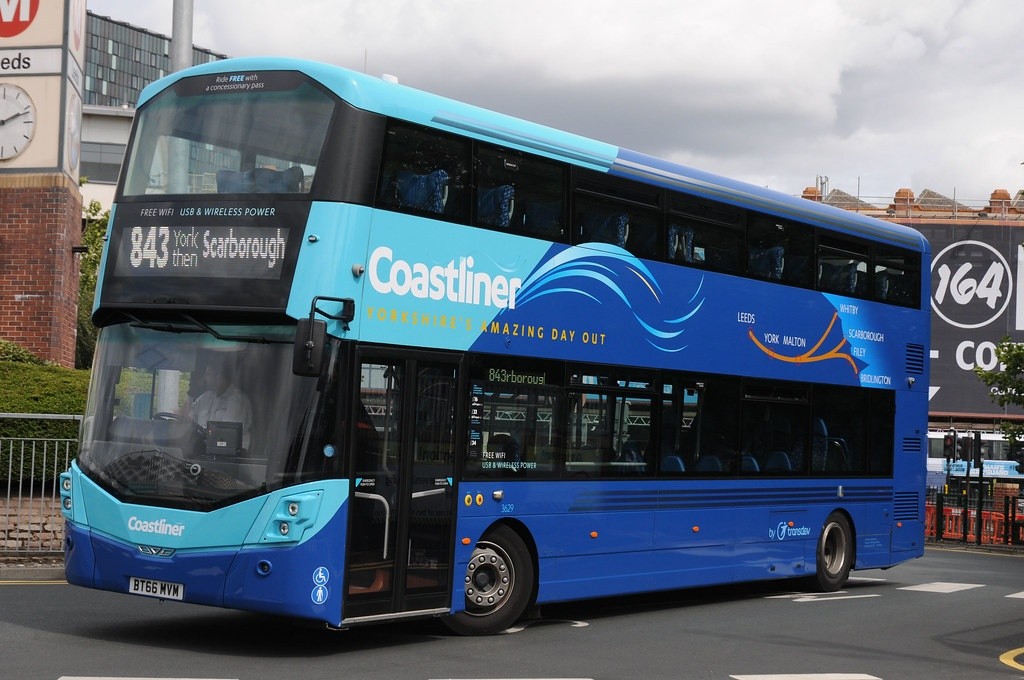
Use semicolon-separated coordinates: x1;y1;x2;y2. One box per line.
52;53;934;635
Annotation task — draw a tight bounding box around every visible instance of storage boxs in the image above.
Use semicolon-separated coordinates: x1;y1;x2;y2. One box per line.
992;483;1019;535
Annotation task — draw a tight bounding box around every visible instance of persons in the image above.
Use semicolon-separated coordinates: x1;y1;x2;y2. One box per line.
173;361;253;450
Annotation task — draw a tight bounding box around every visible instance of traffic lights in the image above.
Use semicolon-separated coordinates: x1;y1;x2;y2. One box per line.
974;432;986;469
943;434;957;459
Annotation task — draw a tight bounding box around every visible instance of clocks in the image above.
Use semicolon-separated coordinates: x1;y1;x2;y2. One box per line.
0;82;37;162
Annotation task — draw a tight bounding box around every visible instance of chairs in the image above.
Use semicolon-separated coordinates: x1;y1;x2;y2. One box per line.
214;166;908;308
487;432;854;477
